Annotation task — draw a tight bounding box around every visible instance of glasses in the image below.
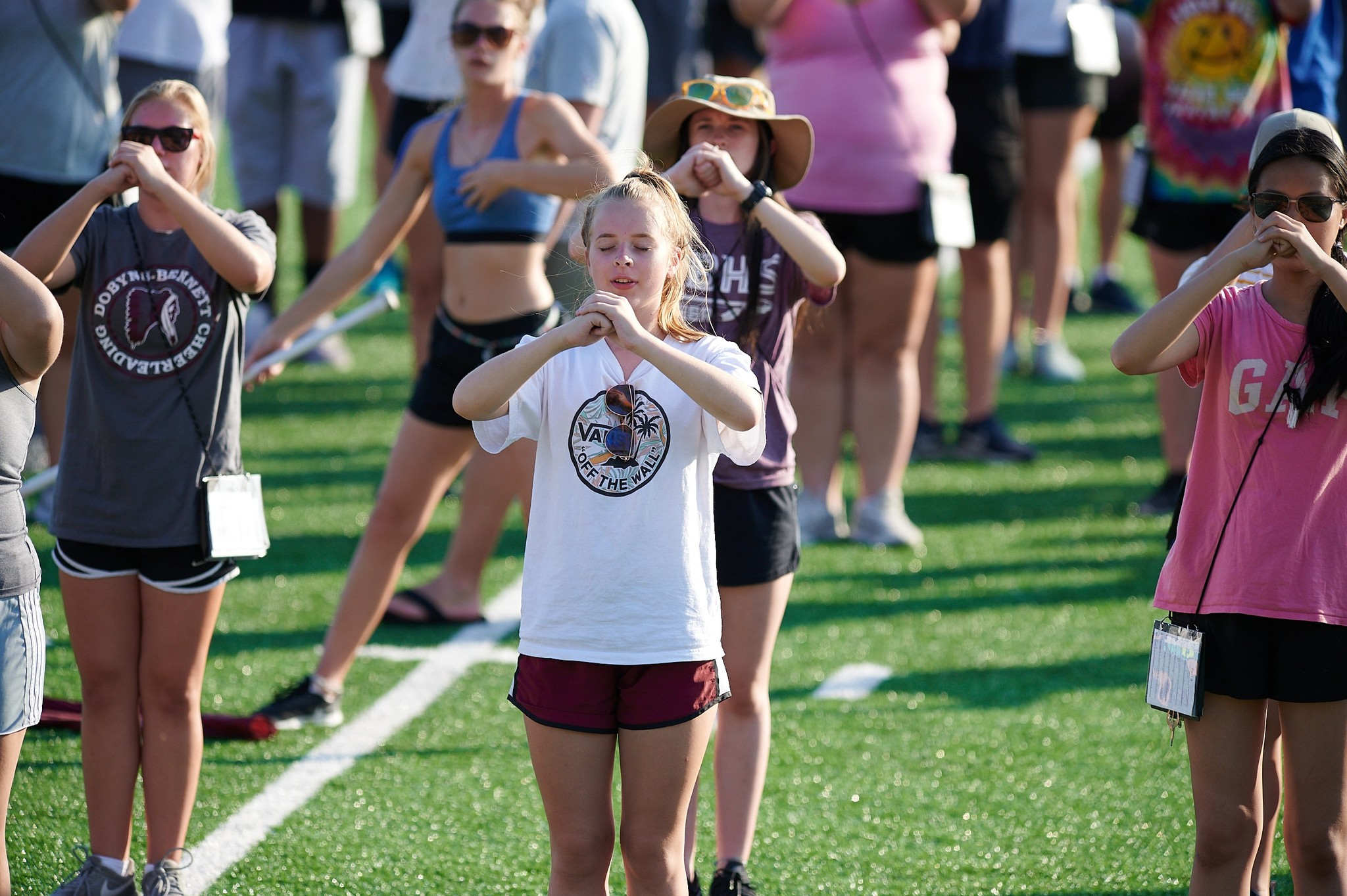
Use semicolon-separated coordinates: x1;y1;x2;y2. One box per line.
121;125;201;152
605;378;639;460
451;20;519;52
682;79;767;109
1249;192;1342;222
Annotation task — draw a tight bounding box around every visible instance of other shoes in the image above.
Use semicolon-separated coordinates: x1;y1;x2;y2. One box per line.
1086;277;1143;317
239;299;349;366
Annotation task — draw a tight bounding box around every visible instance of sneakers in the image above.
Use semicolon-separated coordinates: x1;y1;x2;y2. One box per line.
51;857;137;896
142;860;182;896
852;492;924;550
795;492;836;546
254;676;343;730
1032;340;1087;384
909;421;946;464
960;419;1035;463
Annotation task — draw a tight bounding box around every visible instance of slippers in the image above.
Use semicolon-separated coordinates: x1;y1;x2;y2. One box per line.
381;589;486;625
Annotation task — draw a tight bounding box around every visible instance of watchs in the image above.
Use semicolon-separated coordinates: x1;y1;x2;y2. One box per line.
740;180;773;212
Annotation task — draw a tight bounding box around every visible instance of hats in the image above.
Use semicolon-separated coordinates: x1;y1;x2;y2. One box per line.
643;73;815;191
1249;108;1344;170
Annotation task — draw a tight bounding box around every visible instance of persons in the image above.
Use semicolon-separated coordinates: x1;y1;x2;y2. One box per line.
0;1;1347;896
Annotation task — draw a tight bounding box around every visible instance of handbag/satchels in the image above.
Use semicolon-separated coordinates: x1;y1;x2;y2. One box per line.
1122;144;1155;210
